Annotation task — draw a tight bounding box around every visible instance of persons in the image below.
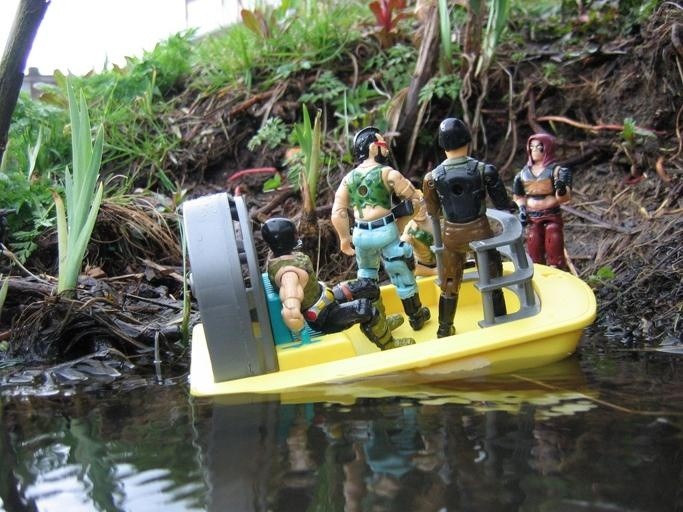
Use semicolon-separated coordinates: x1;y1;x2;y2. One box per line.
261;217;416;351
511;133;574;270
421;117;521;339
330;125;431;331
